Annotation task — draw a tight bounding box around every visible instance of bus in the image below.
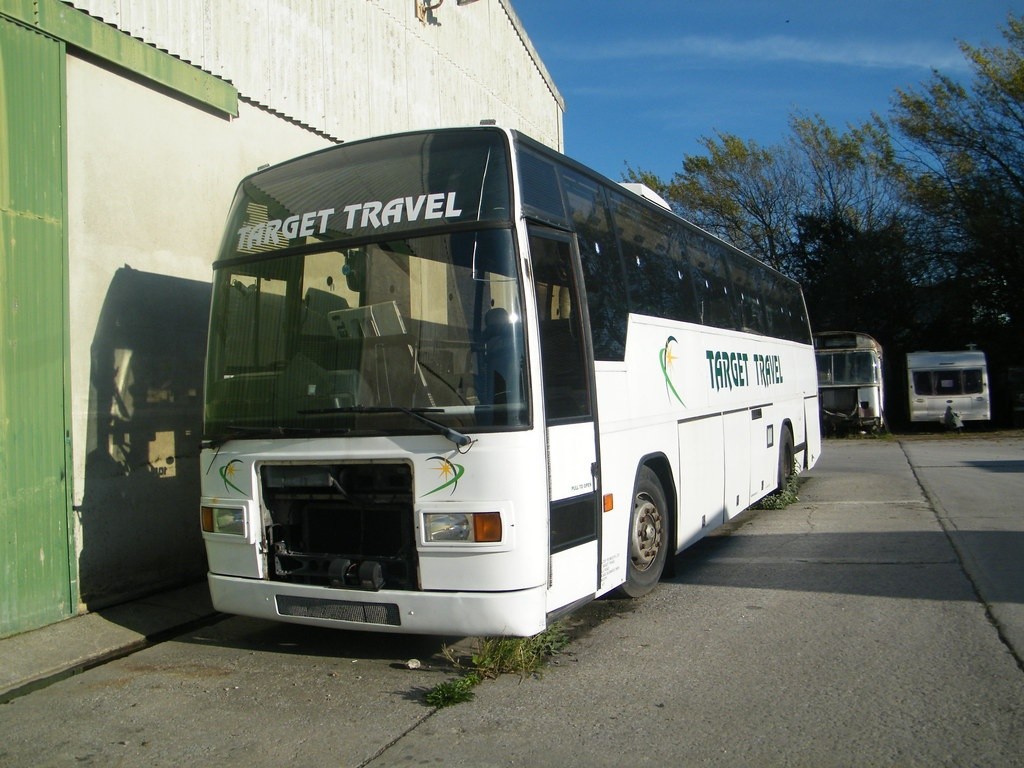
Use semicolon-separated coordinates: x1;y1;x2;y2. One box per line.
196;121;822;639
809;331;887;434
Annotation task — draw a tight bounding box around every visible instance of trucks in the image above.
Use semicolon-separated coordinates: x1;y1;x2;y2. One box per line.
905;350;992;421
